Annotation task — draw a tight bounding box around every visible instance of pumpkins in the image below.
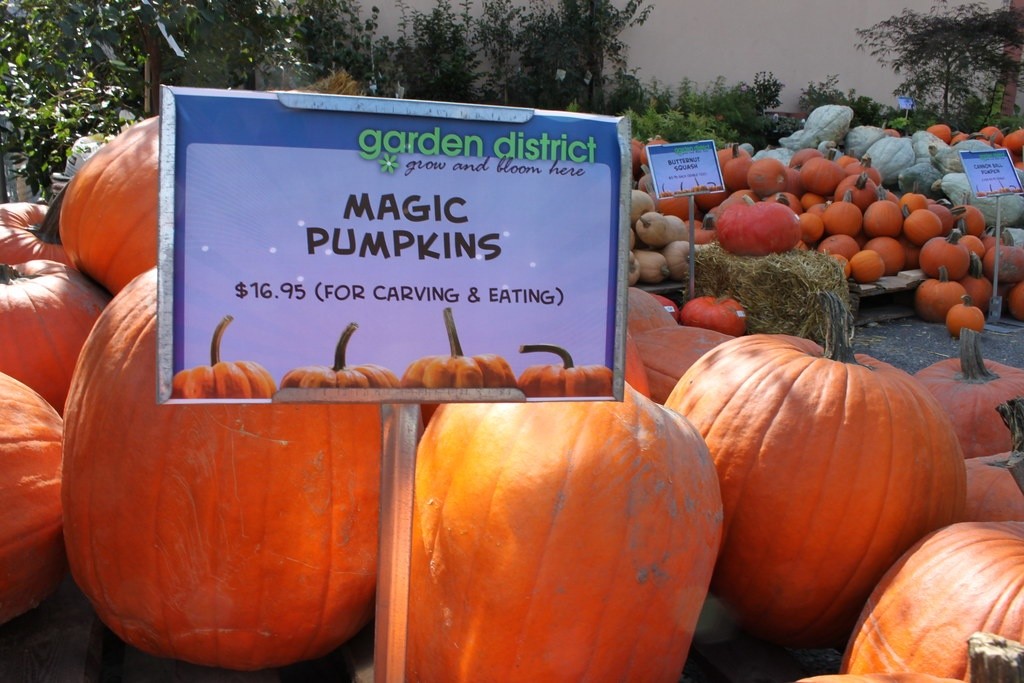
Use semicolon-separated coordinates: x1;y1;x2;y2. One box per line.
0;103;1023;683
